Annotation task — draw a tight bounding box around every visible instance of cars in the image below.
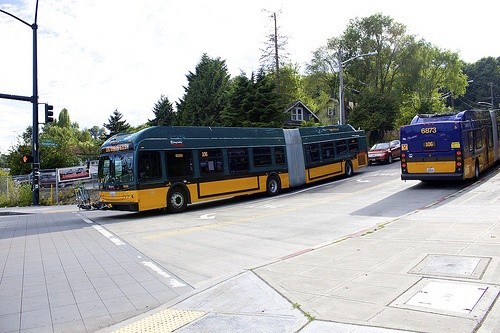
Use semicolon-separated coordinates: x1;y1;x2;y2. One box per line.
368;139;401;164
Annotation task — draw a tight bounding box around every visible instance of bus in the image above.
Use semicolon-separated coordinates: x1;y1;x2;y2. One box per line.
98;124;370;215
398;106;500;185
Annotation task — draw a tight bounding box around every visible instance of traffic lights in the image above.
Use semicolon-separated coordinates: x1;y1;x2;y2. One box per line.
22;154;34;162
44;103;54;123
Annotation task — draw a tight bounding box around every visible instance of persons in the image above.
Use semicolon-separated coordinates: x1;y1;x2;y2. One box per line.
124;166;133;176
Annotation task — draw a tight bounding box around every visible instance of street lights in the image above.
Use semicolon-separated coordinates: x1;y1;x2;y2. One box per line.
337;50;378;125
